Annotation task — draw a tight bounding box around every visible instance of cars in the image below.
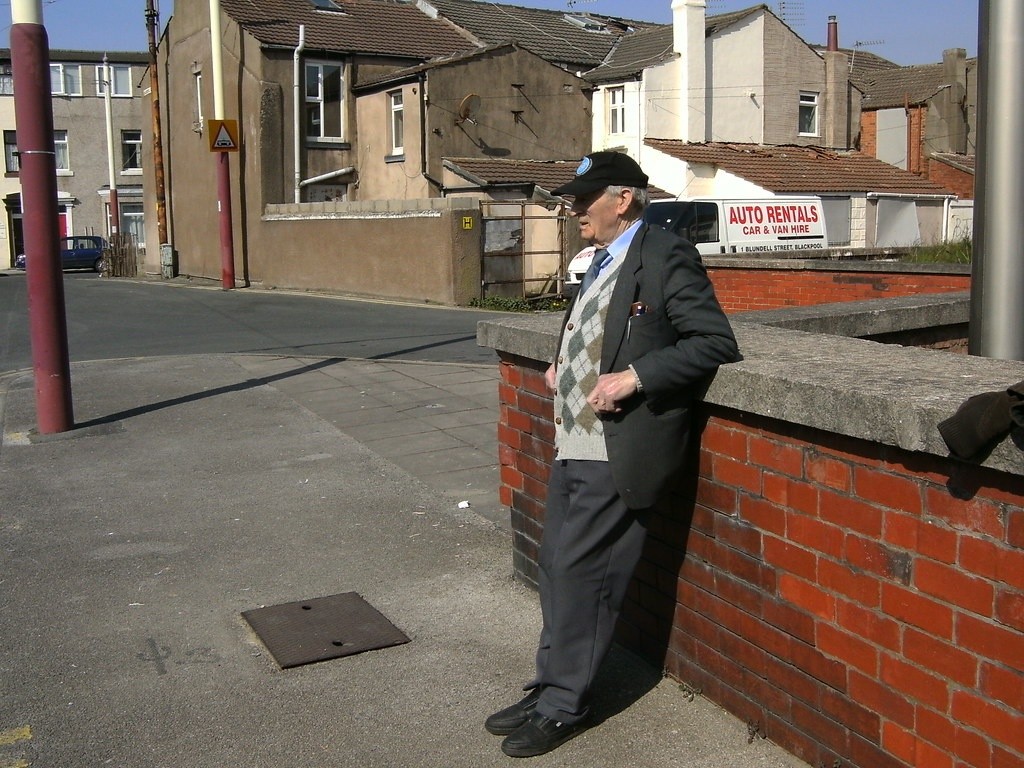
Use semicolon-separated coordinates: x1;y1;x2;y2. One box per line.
15;236;112;272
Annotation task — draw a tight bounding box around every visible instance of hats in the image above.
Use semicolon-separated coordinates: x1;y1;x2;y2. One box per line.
550;151;649;196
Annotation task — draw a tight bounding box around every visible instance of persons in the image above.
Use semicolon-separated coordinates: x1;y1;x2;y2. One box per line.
484;151;738;757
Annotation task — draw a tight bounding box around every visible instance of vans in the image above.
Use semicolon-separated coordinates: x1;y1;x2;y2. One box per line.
562;195;829;298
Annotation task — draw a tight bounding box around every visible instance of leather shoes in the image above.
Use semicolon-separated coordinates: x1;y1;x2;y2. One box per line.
501;711;595;757
484;688;543;735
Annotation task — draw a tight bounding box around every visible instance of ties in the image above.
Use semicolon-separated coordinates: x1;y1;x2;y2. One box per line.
579;250;610;301
